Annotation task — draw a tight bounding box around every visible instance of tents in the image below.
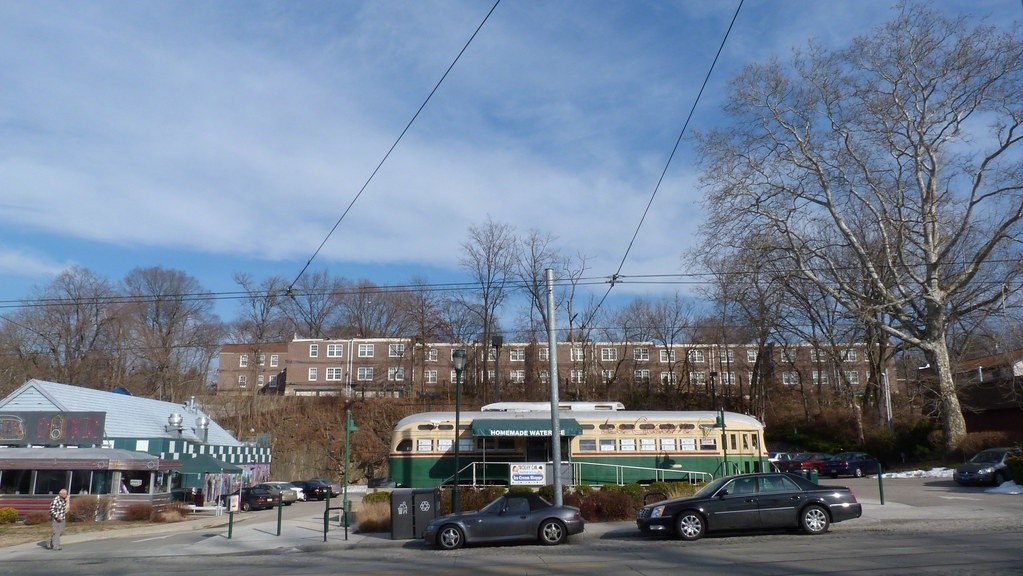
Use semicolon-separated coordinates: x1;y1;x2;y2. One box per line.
175;454;244;507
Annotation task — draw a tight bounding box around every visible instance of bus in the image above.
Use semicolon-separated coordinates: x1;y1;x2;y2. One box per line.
388;400;768;487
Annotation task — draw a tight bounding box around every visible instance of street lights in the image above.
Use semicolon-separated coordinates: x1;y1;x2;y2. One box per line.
710;371;719;404
490;335;504;402
1002;283;1009;325
881;372;891;431
713;400;729;475
342;408;360;526
452;348;465;513
979;366;985;383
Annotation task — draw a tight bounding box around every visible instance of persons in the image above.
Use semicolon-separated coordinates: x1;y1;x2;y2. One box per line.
120;477;130;493
46;489;68;550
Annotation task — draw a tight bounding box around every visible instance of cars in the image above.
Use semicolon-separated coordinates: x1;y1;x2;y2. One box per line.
785;452;838;476
215;487;278;511
253;481;297;506
322;480;343;497
421;491;586;549
636;472;861;542
826;452;886;478
286;482;306;502
952;446;1023;487
292;479;332;501
767;450;792;467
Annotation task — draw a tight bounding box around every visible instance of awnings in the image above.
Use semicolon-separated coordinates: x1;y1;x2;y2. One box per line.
472;419;583;484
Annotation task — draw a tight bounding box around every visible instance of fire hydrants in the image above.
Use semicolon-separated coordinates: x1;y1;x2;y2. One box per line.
347;501;353;518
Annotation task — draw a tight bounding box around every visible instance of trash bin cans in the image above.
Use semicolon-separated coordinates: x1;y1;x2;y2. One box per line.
390;488;441;540
794;469;818;485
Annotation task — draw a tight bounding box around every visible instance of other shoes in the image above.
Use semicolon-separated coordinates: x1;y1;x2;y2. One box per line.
47;544;52;549
55;546;62;550
